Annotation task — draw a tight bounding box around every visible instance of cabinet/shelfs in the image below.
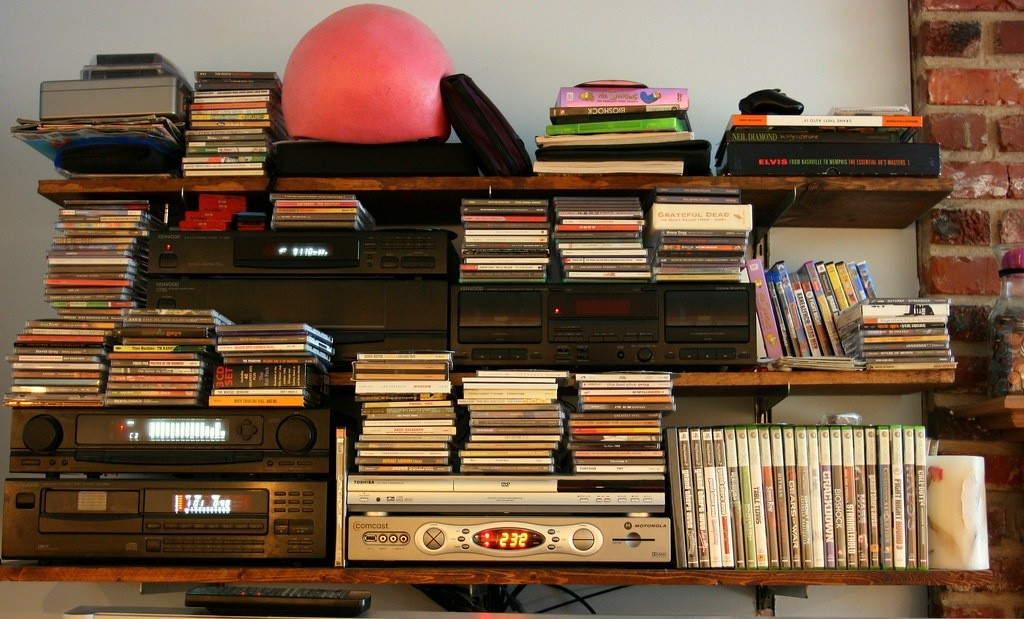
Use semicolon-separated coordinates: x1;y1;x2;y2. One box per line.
1;176;995;588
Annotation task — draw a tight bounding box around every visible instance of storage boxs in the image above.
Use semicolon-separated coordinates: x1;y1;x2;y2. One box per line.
712;142;941;183
37;76;190;121
213;365;331;394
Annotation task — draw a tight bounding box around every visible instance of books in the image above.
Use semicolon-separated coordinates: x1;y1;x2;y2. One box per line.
532;88;711;177
715;114;924;164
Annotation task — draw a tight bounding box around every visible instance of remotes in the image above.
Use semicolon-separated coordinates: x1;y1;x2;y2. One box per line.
184;584;374;617
